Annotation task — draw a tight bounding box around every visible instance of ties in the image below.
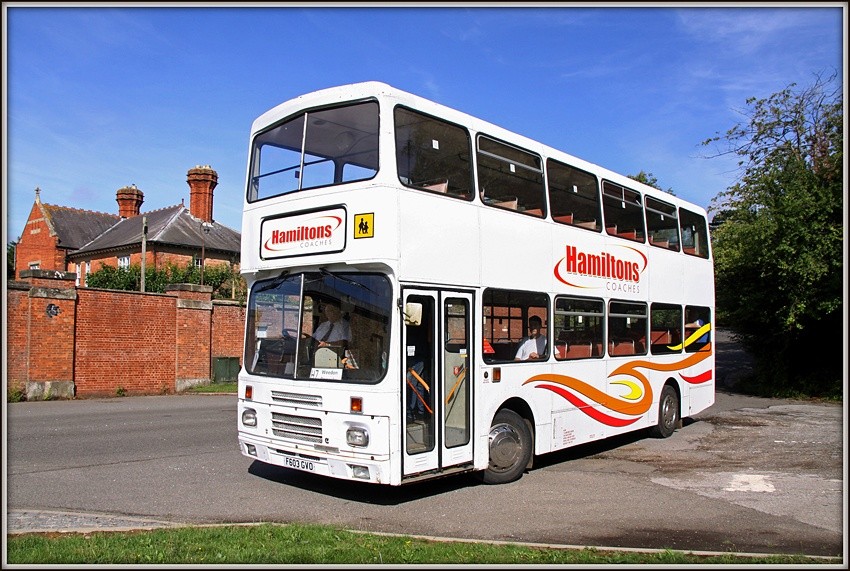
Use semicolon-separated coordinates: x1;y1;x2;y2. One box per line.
321;324;335;342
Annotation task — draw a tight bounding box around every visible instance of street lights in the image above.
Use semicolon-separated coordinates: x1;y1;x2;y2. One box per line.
199;220;210;286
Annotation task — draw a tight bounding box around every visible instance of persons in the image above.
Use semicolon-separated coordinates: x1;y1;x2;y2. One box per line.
311;303;353;348
514;315;561;360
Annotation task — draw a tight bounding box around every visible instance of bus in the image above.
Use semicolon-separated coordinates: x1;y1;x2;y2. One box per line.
236;81;716;487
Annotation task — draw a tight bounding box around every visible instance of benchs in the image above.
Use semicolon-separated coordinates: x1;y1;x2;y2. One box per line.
444;328;695;359
408;175;697;256
